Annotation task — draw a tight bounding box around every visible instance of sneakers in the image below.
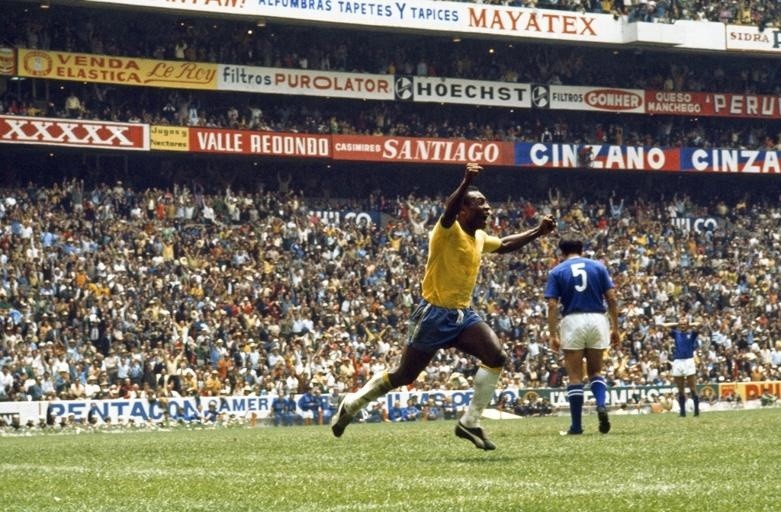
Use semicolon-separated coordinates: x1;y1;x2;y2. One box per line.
330;392;353;438
454;419;497;451
678;410;700;417
567;428;583;435
597;407;611;434
